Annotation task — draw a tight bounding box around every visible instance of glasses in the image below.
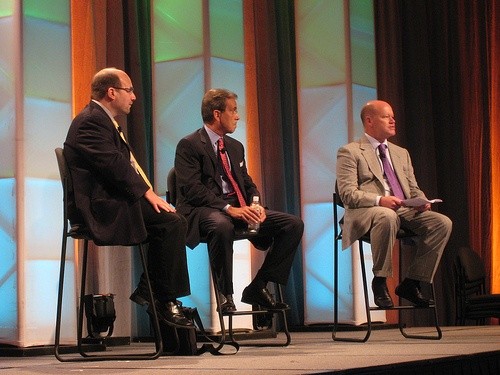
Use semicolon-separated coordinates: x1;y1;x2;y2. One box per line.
109;85;134;94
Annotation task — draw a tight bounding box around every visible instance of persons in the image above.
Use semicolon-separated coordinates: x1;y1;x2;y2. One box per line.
336;100;453;308
174;88;304;313
63;68;196;329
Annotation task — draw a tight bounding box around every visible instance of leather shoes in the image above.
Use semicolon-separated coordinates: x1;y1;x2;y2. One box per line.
371;279;395;307
394;281;435;305
241;286;288;311
216;294;236;312
146;297;194;328
129;286;153;308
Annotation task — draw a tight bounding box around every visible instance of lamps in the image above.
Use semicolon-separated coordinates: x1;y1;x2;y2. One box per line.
85;287;118;346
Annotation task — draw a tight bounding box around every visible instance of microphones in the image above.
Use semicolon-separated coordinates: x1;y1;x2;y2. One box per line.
380;152;384;157
118;125;122;133
221;148;225;154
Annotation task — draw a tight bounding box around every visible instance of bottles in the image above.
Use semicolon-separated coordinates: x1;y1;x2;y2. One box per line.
248;196;260;233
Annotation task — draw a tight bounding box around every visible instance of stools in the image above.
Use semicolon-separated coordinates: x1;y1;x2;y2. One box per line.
166;166;295;350
49;145;165;365
328;177;444;343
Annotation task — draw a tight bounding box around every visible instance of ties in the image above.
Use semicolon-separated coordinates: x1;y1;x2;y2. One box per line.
377;144;405;202
114;120;153;189
218;138;246;208
